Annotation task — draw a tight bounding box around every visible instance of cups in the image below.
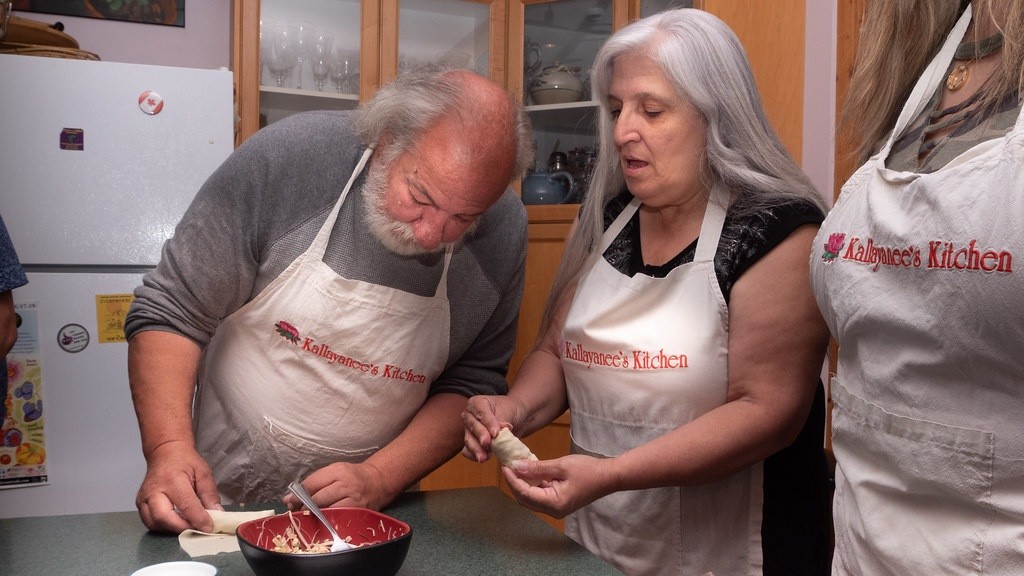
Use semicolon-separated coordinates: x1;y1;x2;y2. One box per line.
523;34;542;105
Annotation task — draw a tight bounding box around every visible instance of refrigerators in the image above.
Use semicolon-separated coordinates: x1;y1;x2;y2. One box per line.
0;53;236;517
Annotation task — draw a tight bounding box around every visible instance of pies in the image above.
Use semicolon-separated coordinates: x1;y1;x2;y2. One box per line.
179;509;275;558
491;427;546;486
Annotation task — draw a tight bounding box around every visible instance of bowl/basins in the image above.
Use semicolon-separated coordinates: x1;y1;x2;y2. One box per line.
236;506;414;576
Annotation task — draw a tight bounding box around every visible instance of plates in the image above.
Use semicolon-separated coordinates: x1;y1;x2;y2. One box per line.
131;561;221;576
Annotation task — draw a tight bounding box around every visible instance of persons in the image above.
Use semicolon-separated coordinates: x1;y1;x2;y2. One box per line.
125;66;531;530
808;0;1024;576
0;213;30;428
462;8;829;575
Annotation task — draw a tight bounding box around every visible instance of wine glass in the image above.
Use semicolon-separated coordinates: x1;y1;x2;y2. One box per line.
257;19;359;94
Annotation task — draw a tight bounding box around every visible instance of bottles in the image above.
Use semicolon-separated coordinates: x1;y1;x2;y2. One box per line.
552;147;597;202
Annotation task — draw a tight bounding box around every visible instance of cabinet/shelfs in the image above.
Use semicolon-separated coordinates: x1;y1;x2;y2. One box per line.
231;0;508;487
508;0;806;533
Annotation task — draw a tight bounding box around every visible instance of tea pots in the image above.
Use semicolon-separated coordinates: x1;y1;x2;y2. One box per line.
529;61;584;106
520;171;576;206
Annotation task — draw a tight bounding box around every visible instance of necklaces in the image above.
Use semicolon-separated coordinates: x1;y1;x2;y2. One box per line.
946;38;986;90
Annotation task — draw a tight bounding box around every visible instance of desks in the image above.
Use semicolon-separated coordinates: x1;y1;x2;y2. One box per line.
0;484;625;576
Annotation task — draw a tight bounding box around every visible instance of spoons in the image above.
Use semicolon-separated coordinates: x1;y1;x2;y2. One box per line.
288;480;353;553
